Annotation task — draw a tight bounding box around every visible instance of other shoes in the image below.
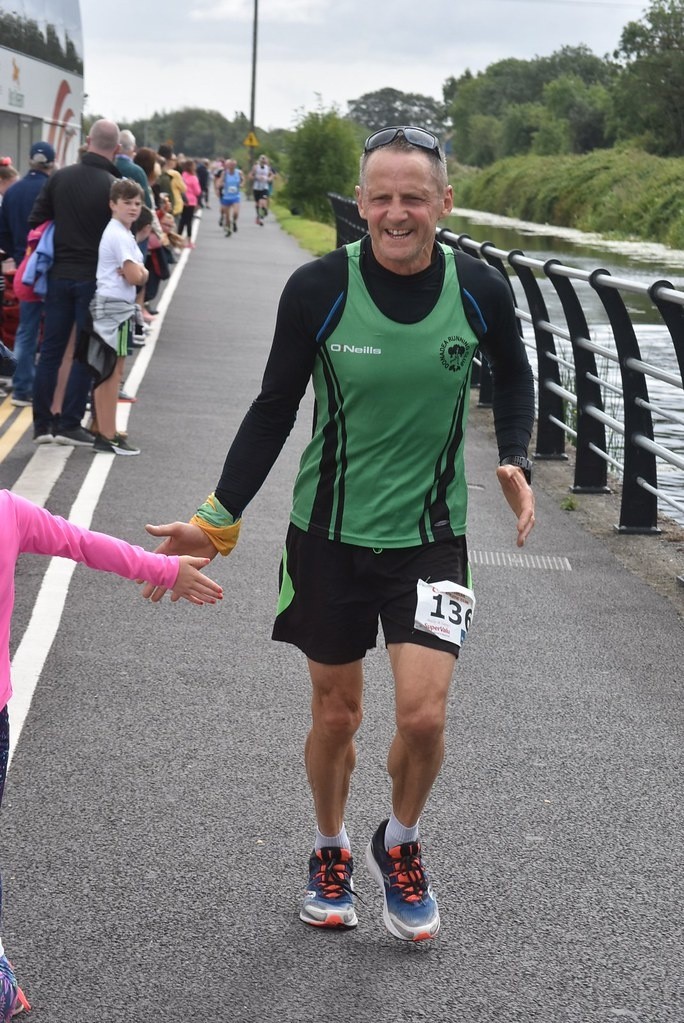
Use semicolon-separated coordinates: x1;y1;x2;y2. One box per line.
118;391;136;403
0;953;31;1023
181;242;195;249
10;393;34;405
132;300;159;346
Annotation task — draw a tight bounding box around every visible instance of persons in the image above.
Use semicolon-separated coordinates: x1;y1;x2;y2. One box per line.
248;154;277;226
136;125;535;943
0;488;224;1023
213;158;246;237
0;118;210;455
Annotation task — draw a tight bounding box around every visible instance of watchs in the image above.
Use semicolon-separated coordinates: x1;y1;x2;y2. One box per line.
501;455;534;484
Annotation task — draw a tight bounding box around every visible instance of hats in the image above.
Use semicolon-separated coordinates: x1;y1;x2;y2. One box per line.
29;141;56;166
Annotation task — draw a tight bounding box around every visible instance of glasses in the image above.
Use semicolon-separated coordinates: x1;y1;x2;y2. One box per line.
364;126;445;167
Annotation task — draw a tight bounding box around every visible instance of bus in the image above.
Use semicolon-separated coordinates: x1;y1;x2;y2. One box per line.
0;0;85;177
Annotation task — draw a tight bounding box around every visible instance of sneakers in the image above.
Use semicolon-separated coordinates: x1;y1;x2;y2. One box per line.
54;427;96;446
366;817;440;941
93;432;140;455
34;425;56;444
300;847;366;929
